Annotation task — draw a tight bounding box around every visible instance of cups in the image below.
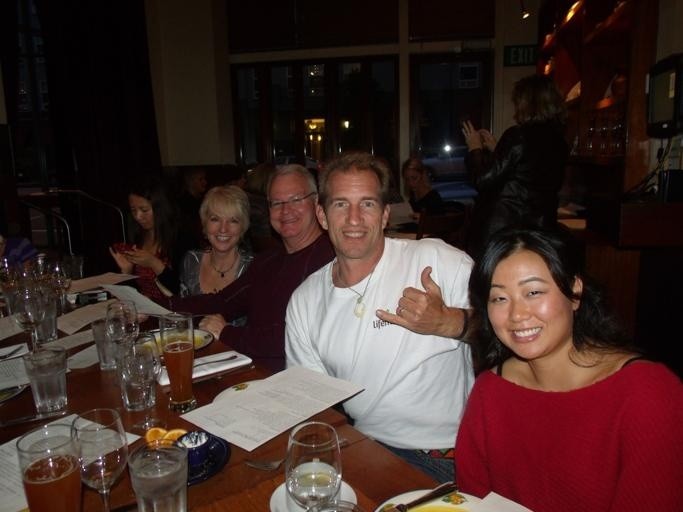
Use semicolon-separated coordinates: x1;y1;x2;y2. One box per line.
0;251;71;351
125;439;189;512
90;299;158;414
17;422;83;512
158;312;197;413
22;349;69;417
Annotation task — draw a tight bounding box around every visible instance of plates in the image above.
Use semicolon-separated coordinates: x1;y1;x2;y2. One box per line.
269;475;357;512
375;486;493;512
135;327;214;354
134;427;228;486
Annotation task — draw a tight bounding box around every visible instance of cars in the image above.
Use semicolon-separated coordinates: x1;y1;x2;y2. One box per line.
419;145;469;179
270;154;323;172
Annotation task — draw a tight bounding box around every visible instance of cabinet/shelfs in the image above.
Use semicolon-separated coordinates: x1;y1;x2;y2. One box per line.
536;0;659;241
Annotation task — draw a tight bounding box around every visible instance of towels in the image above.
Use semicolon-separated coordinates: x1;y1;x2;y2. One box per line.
154;348;253;388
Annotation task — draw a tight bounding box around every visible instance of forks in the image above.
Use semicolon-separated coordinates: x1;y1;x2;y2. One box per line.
375;482;461;511
245;438;347;471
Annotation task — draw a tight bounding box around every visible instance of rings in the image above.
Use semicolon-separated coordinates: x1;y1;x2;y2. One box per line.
398;307;405;314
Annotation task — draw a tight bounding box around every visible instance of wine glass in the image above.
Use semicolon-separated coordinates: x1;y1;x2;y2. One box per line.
72;410;132;512
284;421;344;511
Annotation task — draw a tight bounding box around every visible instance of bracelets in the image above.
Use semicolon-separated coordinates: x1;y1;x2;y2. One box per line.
457;306;470;342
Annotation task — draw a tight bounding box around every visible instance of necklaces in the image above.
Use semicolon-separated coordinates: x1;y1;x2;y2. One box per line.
335;263;374;317
207;253;238;278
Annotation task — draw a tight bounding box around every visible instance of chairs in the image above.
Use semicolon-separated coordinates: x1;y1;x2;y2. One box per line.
416;200;472;251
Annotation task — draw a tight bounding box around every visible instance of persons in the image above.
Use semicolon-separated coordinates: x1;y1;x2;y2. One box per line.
110;180;183;311
401;157;444;231
180;158;275;252
175;185;254;332
455;231;682;512
285;151;476;483
128;164;333;372
460;74;569;249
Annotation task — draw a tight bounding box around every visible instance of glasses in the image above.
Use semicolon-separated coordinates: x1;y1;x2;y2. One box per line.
269;191;315;211
